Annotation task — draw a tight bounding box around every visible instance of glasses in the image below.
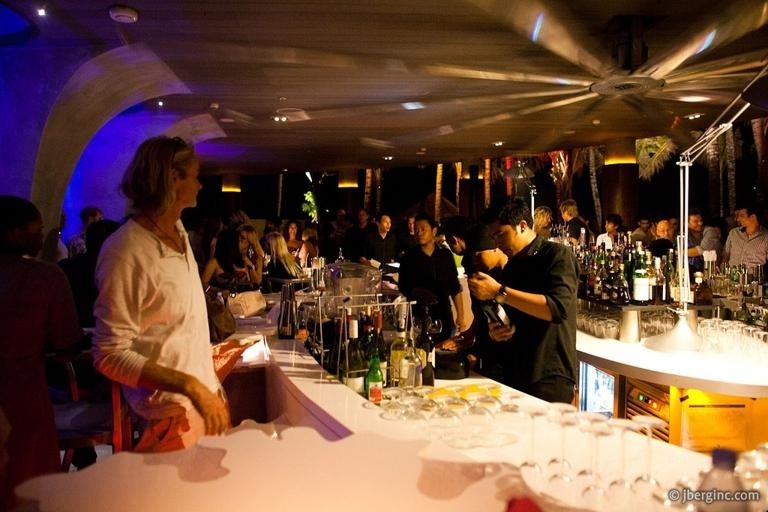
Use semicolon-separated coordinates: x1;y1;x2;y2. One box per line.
170;135;186;169
90;214;103;221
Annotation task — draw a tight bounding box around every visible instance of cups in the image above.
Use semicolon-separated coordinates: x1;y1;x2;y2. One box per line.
697;318;768;357
576;300;675;340
735;442;768;512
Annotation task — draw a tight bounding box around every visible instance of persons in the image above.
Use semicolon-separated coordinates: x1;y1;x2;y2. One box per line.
466;199;581;404
43;198;766;335
90;136;229;451
442;215;485;316
433;213;512;383
397;213;468;350
0;197;84;512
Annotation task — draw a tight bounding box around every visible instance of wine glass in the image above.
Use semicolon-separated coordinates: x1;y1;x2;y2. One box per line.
427;318;443;349
380;384;666;512
744;303;767;328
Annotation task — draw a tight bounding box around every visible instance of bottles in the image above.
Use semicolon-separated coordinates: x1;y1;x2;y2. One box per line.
696;447;742;512
336;247;345;263
568;227;682;306
482;300;512;331
278;282;299;340
308;301;434;406
692;249;763;304
311;255;326;291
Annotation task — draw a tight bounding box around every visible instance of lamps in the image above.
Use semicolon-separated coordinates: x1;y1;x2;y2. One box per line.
645;62;768;354
